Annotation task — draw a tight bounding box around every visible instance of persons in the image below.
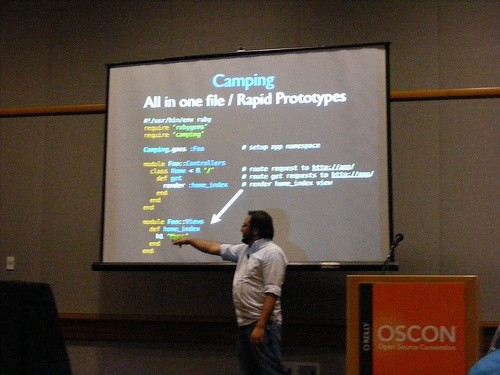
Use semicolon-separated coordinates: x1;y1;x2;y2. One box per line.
171;209;289;375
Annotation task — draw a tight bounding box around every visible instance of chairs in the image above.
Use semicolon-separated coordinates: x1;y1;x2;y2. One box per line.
0;278;72;375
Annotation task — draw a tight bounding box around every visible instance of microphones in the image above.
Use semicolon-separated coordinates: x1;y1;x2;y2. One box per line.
391;233;404;249
